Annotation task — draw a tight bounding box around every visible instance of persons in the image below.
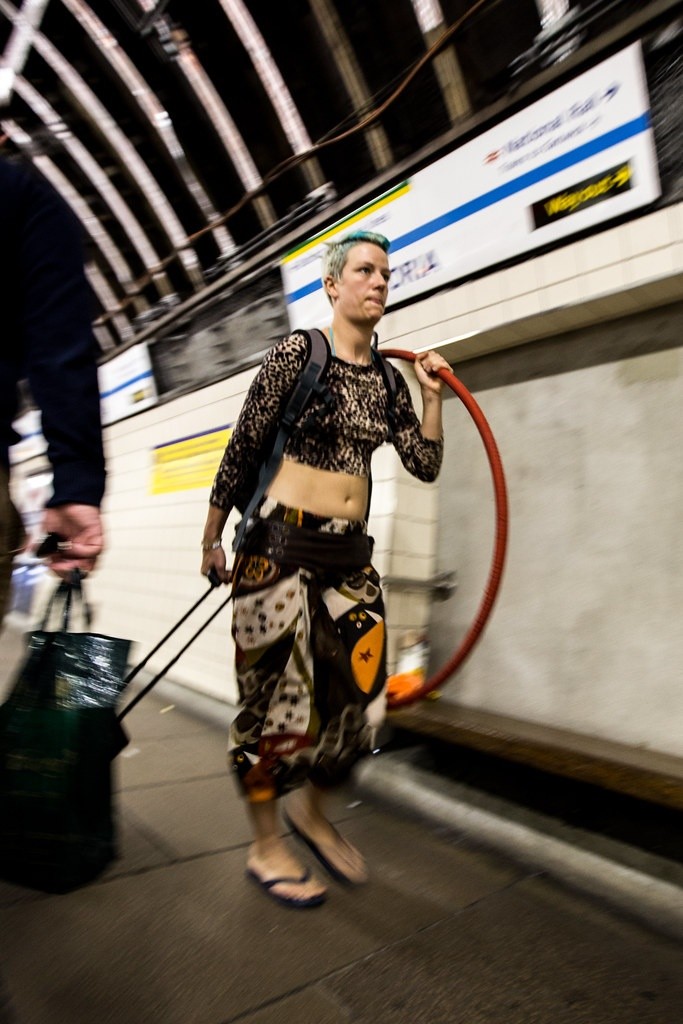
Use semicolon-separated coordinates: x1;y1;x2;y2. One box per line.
0;114;109;639
201;229;452;906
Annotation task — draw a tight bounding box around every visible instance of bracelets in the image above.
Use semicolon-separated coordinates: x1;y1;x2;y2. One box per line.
199;538;220;549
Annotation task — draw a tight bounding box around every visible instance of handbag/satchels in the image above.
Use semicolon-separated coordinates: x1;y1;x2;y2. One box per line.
0;575;131;894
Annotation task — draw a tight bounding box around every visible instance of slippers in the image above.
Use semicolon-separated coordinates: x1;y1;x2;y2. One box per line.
245;846;327;906
280;795;368;887
